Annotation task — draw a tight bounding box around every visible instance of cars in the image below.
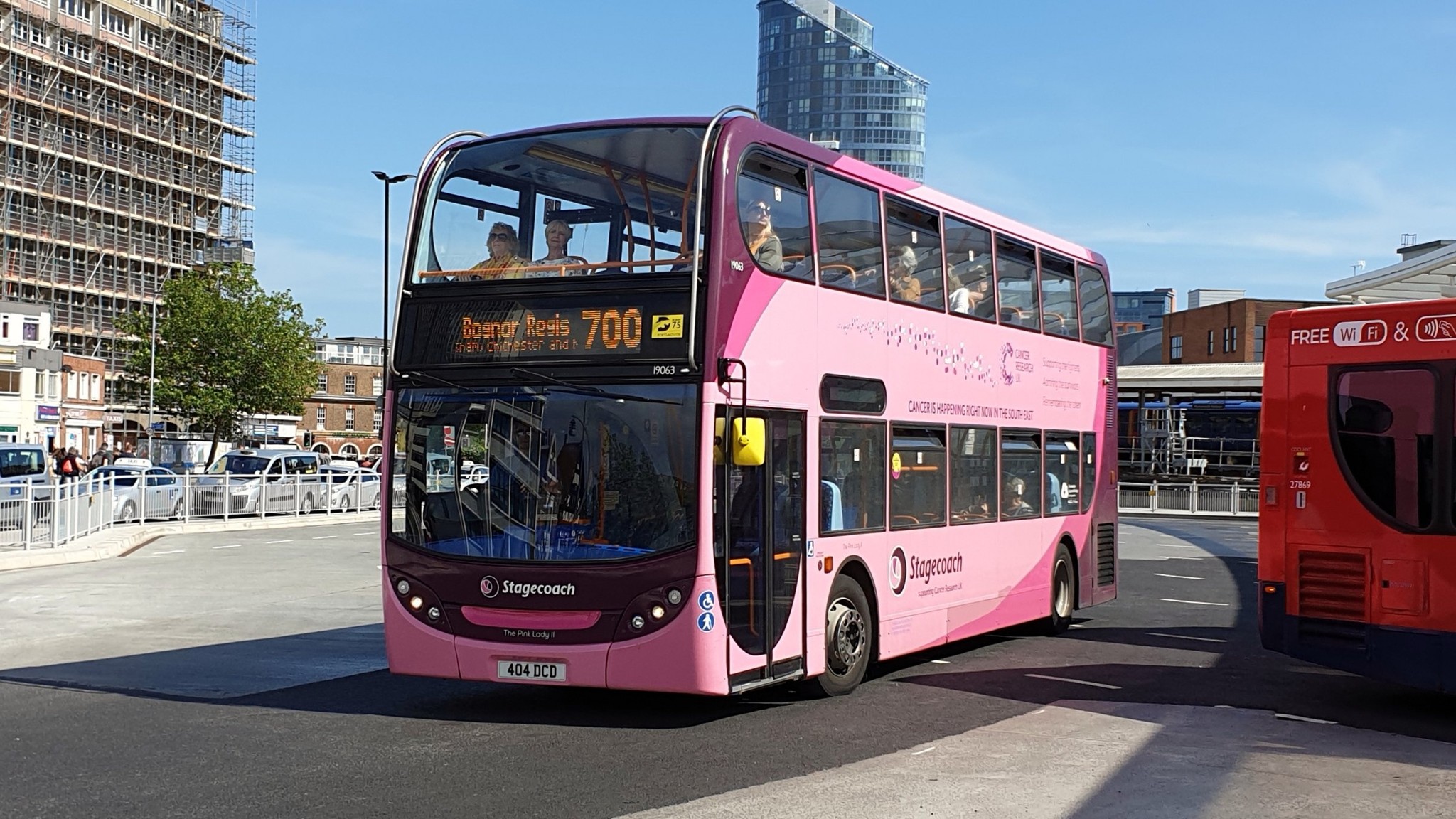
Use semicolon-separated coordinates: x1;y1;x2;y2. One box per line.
56;457;193;525
424;450;490;494
318;453;408;513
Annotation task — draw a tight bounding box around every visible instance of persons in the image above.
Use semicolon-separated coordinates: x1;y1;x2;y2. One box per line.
233;461;274;481
933;263;993;315
688;195;783;273
865;245;920;303
828;461;844;477
52;442;137;490
484;414;561;505
981;477;1034;516
466;469;478;480
525;219;587;278
361;457;372;467
457;221;529;281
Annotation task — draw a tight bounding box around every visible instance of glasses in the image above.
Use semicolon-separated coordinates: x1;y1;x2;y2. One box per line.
489;233;511;242
513;430;533;438
746;206;773;216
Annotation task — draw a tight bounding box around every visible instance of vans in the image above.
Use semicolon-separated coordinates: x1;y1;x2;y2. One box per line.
0;443;52;529
192;448;322;517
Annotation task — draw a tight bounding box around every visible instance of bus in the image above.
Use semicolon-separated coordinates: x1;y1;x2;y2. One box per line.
1257;297;1456;695
377;104;1116;699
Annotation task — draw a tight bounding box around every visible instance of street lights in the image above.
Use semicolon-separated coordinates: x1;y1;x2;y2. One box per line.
371;169;417;440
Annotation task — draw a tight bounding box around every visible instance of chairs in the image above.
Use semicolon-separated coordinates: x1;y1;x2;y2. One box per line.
18;455;32;469
566;256;1071;333
891;510;1012;524
